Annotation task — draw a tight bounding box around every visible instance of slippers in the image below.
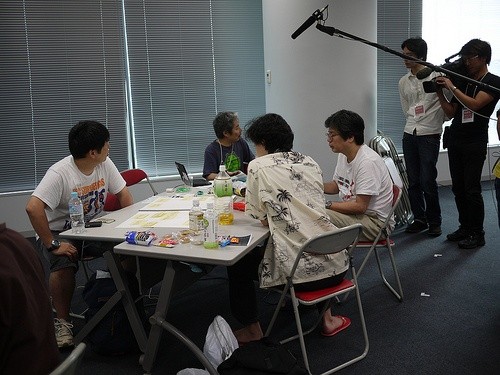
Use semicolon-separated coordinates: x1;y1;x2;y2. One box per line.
321;315;351;337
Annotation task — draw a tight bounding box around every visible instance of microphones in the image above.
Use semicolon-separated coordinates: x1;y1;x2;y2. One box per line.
290;16;316;39
415;67;431;79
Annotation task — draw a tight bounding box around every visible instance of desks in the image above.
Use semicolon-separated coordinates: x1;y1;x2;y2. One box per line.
59;180;271;375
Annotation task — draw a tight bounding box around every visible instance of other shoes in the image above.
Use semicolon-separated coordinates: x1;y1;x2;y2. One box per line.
327;130;340;137
428;228;443;235
459;237;486;249
406;221;429;233
447;228;472;241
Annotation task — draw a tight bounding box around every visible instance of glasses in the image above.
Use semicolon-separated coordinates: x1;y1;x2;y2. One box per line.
464;55;479;65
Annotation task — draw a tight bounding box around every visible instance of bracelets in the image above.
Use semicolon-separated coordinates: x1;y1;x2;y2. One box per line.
452;87;456;91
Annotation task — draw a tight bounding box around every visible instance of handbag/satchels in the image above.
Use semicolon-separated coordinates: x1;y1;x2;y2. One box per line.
217;336;309;375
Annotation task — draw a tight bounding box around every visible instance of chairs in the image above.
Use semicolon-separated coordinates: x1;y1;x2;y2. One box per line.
370;130;414;226
120;169;158;196
263;223;370;375
349;185;405;301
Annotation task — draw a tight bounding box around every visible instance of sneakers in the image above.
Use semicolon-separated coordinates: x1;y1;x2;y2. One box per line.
52;318;75;348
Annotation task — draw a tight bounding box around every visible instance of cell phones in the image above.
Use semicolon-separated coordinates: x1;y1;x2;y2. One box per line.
84;221;102;228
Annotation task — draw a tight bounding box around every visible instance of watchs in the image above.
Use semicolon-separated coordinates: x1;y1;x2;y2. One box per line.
47;239;60;252
325;200;333;210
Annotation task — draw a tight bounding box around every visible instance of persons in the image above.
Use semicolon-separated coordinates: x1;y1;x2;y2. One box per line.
431;37;500;249
318;110;395;243
398;37;456;236
25;118;139;350
0;222;62;375
202;110;256;182
224;115;353;341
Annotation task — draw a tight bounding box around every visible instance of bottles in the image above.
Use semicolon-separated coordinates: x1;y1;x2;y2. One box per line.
188;199;204;244
214;164;233;224
203;202;219;248
69;192;85;233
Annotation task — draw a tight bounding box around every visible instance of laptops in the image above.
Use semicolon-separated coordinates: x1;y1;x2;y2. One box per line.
175;161;212;187
